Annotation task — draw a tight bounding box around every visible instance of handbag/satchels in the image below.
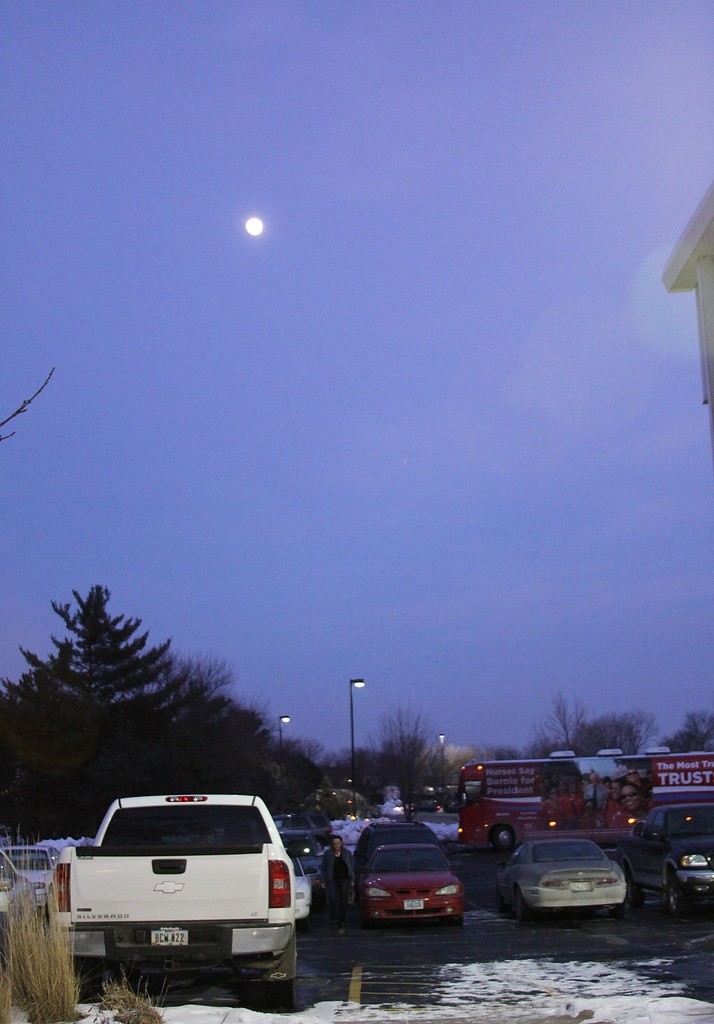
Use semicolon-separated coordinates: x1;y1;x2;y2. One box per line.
346;887;355;904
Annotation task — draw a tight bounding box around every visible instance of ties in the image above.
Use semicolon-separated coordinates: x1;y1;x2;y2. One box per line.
593;786;596;801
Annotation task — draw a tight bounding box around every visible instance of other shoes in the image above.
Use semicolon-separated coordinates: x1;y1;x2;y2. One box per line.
338;929;344;936
331;920;336;923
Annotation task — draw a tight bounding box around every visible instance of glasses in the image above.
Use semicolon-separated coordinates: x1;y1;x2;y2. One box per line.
627;771;637;776
619;792;637;800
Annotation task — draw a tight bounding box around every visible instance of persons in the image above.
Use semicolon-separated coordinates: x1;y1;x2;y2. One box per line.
542;766;654;829
319;835;356;936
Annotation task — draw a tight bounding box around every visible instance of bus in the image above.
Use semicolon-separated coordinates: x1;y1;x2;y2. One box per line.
411;785;478;813
456;745;714;851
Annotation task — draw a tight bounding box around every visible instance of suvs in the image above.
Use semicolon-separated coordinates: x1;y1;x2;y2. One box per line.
353;820;445;895
279;830;331;904
272;811;334;851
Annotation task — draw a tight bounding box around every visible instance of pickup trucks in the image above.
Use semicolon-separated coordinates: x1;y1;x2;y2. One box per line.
616;803;714;916
45;793;296;1011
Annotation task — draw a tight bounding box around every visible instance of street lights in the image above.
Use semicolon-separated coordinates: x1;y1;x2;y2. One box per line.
350;679;365;818
439;733;445;803
280;716;290;809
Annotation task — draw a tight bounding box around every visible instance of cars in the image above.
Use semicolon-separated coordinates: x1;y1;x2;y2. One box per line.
357;843;463;929
433;800;446;814
287;853;318;928
0;848;45;950
3;846;60;922
495;840;627;922
